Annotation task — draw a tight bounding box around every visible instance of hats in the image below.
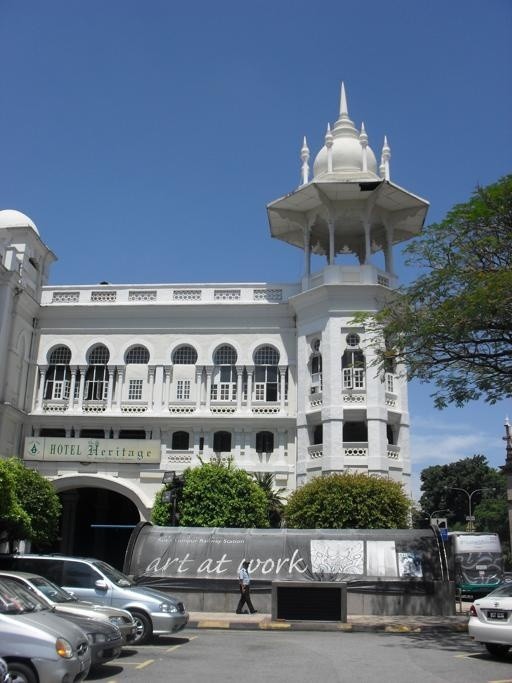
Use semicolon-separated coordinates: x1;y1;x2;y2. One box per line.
243;560;250;564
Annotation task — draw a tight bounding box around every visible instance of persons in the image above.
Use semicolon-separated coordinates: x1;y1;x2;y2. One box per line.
236;561;257;614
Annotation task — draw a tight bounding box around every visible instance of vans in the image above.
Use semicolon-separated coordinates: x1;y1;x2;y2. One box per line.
0;573;93;682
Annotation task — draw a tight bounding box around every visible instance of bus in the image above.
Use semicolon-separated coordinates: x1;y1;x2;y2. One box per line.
444;528;506;601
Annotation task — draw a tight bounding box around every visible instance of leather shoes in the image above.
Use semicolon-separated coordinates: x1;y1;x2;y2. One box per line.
251;609;257;613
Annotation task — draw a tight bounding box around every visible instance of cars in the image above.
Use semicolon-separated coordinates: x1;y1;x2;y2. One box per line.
465;581;512;660
0;567;141;646
0;577;127;667
503;569;512;583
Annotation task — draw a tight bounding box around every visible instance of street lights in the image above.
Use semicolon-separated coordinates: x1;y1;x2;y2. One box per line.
441;485;497;530
157;468;185;526
415;507;452;519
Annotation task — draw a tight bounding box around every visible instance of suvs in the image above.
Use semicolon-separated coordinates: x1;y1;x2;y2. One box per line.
0;549;192;648
